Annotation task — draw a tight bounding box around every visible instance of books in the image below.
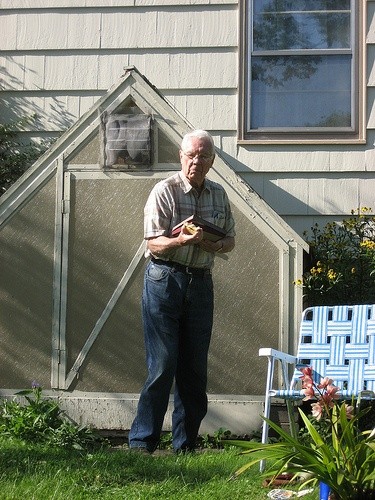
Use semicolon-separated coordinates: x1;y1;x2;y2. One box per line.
172;215;226;242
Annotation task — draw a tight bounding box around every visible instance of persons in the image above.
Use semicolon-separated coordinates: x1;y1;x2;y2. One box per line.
128;129;237;455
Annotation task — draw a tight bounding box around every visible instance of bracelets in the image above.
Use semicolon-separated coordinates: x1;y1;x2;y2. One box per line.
217;240;224;252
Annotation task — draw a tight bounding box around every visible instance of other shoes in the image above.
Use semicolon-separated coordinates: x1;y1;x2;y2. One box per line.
131;447;150;457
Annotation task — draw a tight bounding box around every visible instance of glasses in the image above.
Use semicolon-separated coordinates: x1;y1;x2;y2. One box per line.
182;150;215;162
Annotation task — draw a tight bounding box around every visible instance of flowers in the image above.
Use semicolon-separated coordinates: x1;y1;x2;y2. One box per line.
218;366;375;500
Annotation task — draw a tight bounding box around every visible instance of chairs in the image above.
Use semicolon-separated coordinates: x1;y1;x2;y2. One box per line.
259;304;375;471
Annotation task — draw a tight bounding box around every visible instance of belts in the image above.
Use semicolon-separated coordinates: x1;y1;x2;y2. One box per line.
151;256;210;275
